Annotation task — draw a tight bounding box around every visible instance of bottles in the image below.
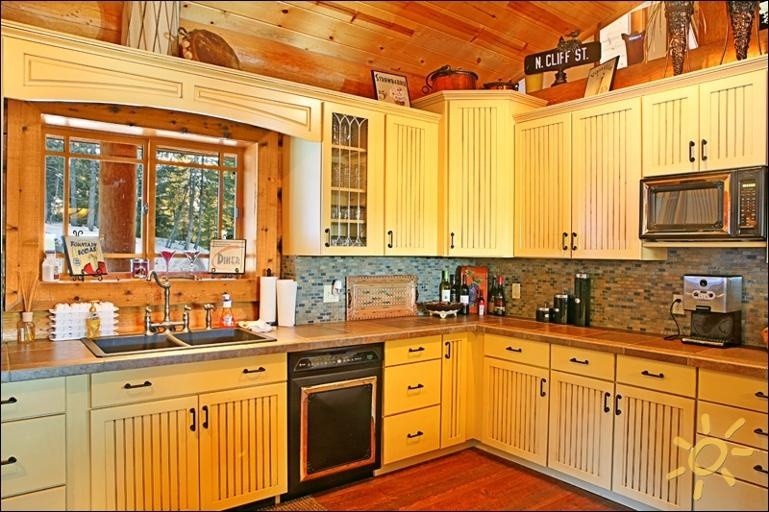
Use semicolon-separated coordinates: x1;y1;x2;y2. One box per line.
52;239;66;280
535;272;590;328
333;119;361;189
438;268;506;317
42;250;60;283
18;310;36;344
219;295;234;327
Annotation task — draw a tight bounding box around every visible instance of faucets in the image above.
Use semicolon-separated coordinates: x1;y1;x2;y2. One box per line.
204;304;216;330
146;270;171;335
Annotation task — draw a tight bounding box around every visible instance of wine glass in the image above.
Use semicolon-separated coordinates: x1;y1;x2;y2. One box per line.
159;249;176;281
183;251;201;277
331;193;365;247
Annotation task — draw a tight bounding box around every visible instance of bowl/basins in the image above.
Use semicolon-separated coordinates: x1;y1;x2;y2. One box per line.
423;301;464;317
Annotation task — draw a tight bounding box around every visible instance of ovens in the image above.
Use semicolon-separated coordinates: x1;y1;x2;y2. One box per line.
286;344;383;499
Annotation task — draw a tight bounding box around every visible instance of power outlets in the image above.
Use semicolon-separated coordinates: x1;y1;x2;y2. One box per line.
672;294;685;316
323;285;341;304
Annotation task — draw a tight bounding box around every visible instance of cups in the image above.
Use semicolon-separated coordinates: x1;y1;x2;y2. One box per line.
132;259;149;278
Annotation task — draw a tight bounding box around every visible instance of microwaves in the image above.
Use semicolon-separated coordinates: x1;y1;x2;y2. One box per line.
639;167;766;243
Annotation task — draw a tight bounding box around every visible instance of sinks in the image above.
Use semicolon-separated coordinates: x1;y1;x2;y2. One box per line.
174;328;267;346
91;335;186;354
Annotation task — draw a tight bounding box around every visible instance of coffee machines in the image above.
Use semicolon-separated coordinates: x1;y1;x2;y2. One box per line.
680;273;744;347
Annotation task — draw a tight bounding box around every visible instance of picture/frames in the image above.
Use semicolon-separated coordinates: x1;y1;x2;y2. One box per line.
208;239;247;278
61;234;108;281
370;70;411;108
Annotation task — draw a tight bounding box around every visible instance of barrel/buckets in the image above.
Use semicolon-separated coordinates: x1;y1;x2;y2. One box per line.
425;65;479;93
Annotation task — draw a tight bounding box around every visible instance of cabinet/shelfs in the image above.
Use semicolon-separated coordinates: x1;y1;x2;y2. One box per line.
641;69;768;176
514;95;642;260
380;332;472;476
282;101;441;255
446;99;509;256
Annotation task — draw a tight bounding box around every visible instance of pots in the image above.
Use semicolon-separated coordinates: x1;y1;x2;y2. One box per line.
431;68;479;93
488;80;520;92
175;27;241;69
